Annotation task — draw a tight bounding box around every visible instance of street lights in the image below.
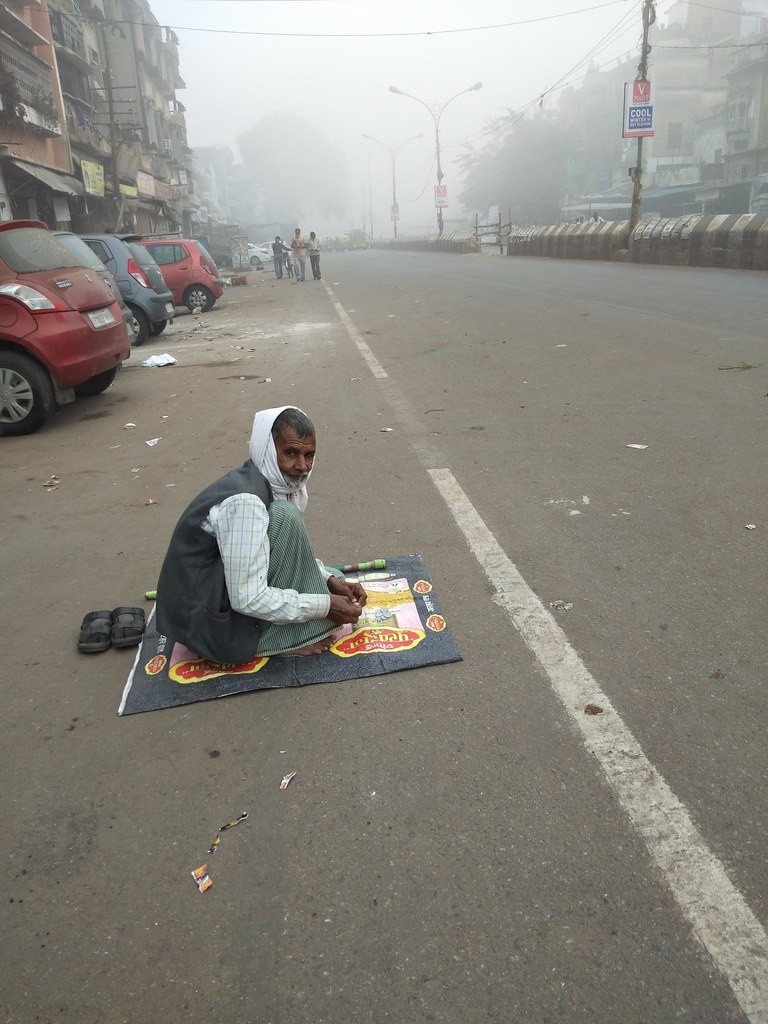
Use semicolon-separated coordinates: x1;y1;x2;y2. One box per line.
388;83;486;239
361;133;428;238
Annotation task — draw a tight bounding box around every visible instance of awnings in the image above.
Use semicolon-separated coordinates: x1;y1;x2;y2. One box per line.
5;158;79;197
642;186;704;199
561;202;632;210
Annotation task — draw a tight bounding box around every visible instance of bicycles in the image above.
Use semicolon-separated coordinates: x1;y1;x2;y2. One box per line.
280;248;295;279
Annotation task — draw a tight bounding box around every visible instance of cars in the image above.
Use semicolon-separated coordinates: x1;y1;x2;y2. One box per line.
77;232;174;347
247;243;272;266
259;242;292;260
0;218;142;437
129;235;223;314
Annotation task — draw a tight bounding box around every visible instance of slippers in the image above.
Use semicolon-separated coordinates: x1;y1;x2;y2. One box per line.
78;610;111;652
111;606;146;648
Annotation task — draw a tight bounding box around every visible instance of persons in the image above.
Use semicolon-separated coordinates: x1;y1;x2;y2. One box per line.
155;406;368;665
507;221;539;230
323;236;345;253
272;228;321;281
560;212;607;225
